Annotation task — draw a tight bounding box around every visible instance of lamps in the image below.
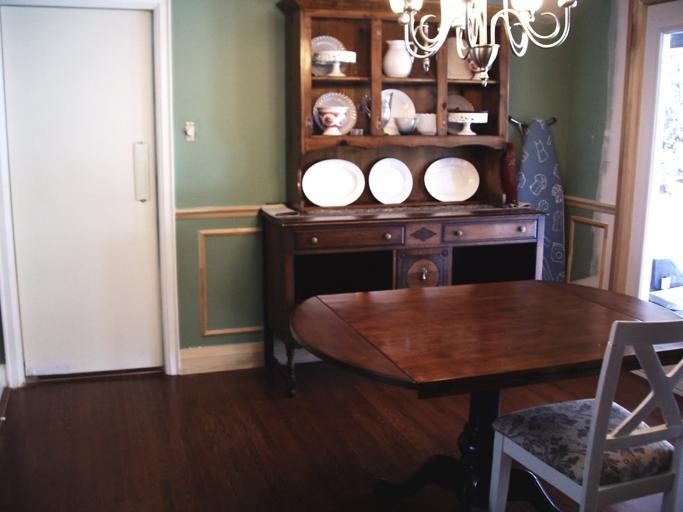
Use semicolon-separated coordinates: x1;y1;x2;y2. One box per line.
389;0;578;88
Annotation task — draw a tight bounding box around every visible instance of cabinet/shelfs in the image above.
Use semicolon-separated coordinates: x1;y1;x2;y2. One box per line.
259;0;545;399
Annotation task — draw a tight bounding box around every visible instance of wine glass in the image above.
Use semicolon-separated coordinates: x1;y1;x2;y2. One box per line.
316;106;351;135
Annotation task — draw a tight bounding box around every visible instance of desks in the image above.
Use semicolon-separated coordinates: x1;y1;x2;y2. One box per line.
288;281;683;510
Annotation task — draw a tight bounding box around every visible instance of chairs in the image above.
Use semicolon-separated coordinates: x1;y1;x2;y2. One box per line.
488;320;683;511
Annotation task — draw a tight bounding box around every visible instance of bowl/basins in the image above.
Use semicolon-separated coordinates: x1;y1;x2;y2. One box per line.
394;113;437;135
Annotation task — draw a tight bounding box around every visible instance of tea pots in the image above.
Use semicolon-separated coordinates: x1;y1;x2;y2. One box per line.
383;39;416;78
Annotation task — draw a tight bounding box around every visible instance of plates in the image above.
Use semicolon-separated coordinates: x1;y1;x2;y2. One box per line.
435;36;475;79
302;159;367;209
449;95;475;134
311;36;349;77
423;156;481;202
381;89;415;135
313;92;359;136
368;157;414;204
